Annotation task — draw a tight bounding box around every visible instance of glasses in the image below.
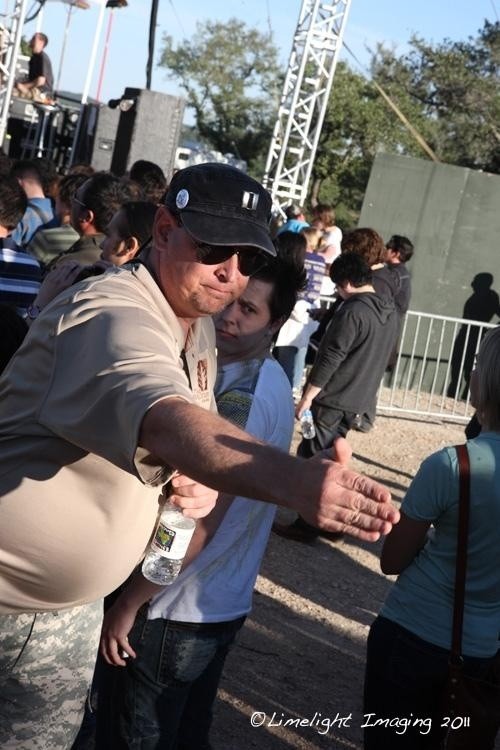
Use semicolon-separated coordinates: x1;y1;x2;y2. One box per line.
72;190;86;208
386;244;393;249
192;242;267;275
311;217;319;224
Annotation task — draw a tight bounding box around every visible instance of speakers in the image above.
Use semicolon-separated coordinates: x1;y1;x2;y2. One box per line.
109;87;186;189
73;104;121;172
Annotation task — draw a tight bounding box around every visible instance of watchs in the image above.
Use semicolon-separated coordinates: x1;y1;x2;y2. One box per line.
24;301;42;321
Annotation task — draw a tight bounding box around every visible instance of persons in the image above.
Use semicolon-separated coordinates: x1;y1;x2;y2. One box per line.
270;251;399;550
100;240;309;749
0;161;402;750
364;321;500;750
0;174;39;315
307;227;402;336
7;164;55;252
311;204;344;296
25;170;132;279
12;33;56;104
274;203;311;238
26;200;162;331
273;227;326;390
310;217;332;258
128;159;167;200
350;236;414;432
25;174;86;266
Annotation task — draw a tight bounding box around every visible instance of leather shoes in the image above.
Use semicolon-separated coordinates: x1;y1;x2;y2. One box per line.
322;529;345;544
271;519;319;547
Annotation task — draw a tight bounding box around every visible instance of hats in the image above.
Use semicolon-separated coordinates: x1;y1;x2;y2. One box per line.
284;204;303;219
164;162;277;258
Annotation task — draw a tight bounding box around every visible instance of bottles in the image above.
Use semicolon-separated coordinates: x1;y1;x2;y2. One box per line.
299;407;315;439
142;494;199;588
